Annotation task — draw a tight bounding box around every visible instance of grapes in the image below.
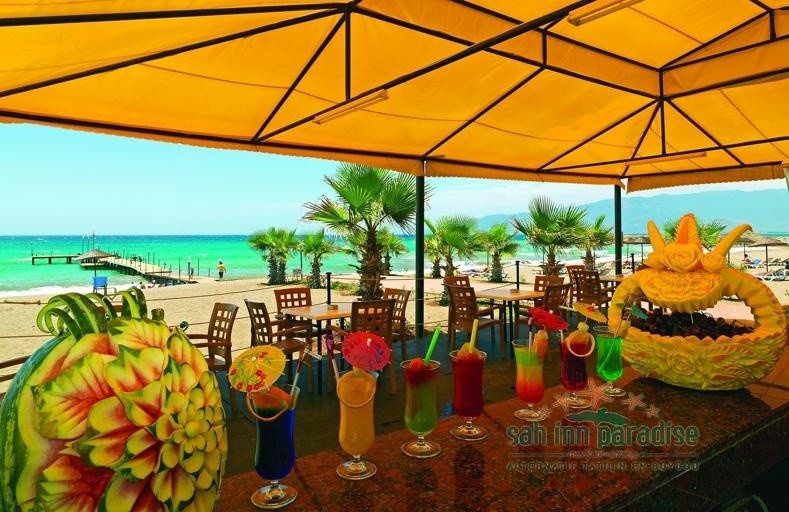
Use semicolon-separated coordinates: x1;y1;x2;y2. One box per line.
628;306;754;340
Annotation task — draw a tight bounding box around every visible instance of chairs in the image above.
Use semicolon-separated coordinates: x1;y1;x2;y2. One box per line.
243;287;411;395
566;266;616;321
744;257;789;284
187;302;238;411
92;275;109;296
515;276;573;364
441;276;506;356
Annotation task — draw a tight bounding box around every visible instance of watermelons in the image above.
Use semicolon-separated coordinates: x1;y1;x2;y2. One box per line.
0;285;229;512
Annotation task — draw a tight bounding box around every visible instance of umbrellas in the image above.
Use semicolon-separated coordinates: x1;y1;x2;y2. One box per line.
613;236;637;260
631;236;651;262
749;236;787;273
734;237;756;255
577;241;607;265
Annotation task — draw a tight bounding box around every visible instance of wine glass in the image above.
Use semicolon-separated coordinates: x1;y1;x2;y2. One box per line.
510;337;548;423
448;348;490;442
556;331;593;411
592;324;627;397
249;383;300;509
334;369;380;482
398;358;443;459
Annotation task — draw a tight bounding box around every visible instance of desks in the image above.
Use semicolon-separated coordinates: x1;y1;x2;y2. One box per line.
600;274;626;296
470;279;544;357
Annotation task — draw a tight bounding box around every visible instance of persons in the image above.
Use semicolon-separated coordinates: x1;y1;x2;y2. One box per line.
217;260;226;278
190;266;196;279
462;266;489;273
744;252;754;269
